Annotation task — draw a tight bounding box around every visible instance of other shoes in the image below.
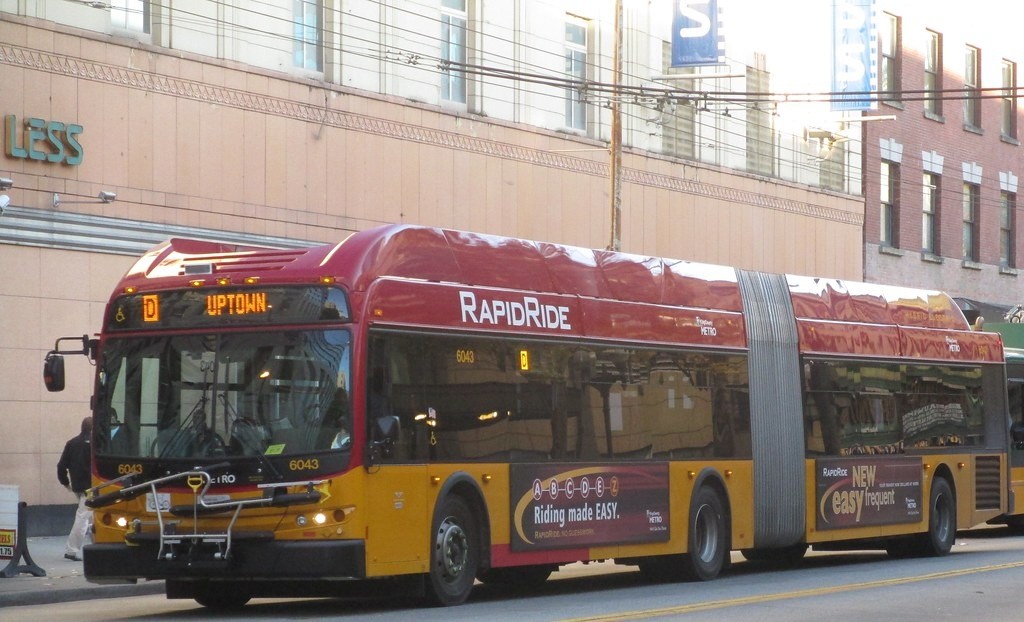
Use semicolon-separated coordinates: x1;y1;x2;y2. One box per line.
64;553;81;561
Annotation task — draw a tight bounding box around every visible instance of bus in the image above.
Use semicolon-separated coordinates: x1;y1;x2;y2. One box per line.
40;226;1023;607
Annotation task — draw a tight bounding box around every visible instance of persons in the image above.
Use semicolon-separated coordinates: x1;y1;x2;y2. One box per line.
110;408;127;453
56;417;94;562
191;410;218;448
